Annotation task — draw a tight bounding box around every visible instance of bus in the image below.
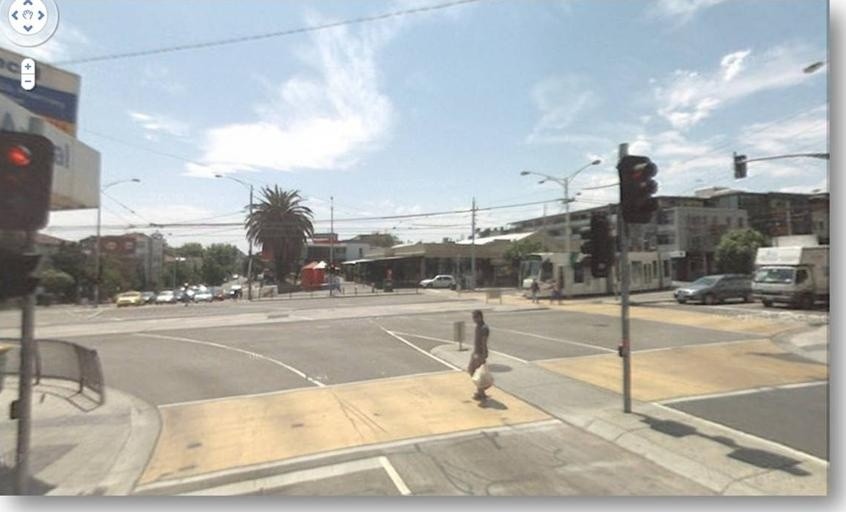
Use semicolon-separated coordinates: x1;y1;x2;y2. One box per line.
522;249;672;300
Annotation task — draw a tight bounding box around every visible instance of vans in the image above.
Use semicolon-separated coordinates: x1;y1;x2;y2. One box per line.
675;273;755;304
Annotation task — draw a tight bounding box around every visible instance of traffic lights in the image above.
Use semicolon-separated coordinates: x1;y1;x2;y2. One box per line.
0;130;53;231
330;262;335;273
0;248;42;301
618;156;659;227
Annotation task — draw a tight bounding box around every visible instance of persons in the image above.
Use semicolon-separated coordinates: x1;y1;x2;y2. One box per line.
544;277;561;304
528;278;540;304
181;289;191;307
464;308;491;408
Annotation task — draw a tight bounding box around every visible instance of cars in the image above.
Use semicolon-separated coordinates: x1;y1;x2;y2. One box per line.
114;279;246;308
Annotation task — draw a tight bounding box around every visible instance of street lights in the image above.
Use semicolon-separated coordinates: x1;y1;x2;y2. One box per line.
326;195;335;297
213;174;254;300
519;158;602;298
813;187;827;193
92;177;141;304
803;62;827;74
166;231;177;290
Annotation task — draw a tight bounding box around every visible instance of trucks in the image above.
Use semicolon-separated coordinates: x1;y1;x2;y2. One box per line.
752;244;831;311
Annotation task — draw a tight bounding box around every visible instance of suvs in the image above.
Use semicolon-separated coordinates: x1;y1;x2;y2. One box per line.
418;274;457;288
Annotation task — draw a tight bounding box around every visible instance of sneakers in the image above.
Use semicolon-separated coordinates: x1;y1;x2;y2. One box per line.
473;393;481;399
479;400;490;407
